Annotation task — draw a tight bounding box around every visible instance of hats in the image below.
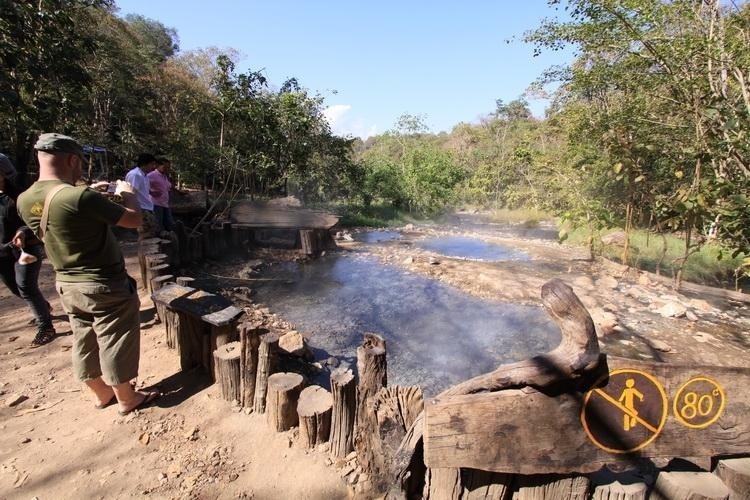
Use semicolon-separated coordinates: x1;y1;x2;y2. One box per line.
33;132;90;165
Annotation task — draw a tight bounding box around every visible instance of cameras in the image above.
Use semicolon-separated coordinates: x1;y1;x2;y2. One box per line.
106;184;117;193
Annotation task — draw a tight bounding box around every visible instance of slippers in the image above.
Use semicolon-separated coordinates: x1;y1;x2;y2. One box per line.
118;386;161;416
94;380;136;409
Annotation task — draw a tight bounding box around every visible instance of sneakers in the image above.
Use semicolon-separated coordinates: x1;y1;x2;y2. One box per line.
30;326;56;346
26;304;53;321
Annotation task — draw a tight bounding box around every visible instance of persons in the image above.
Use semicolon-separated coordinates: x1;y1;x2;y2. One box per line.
148;158;179;231
0;151;57;348
16;131;158;416
124;153;158;237
